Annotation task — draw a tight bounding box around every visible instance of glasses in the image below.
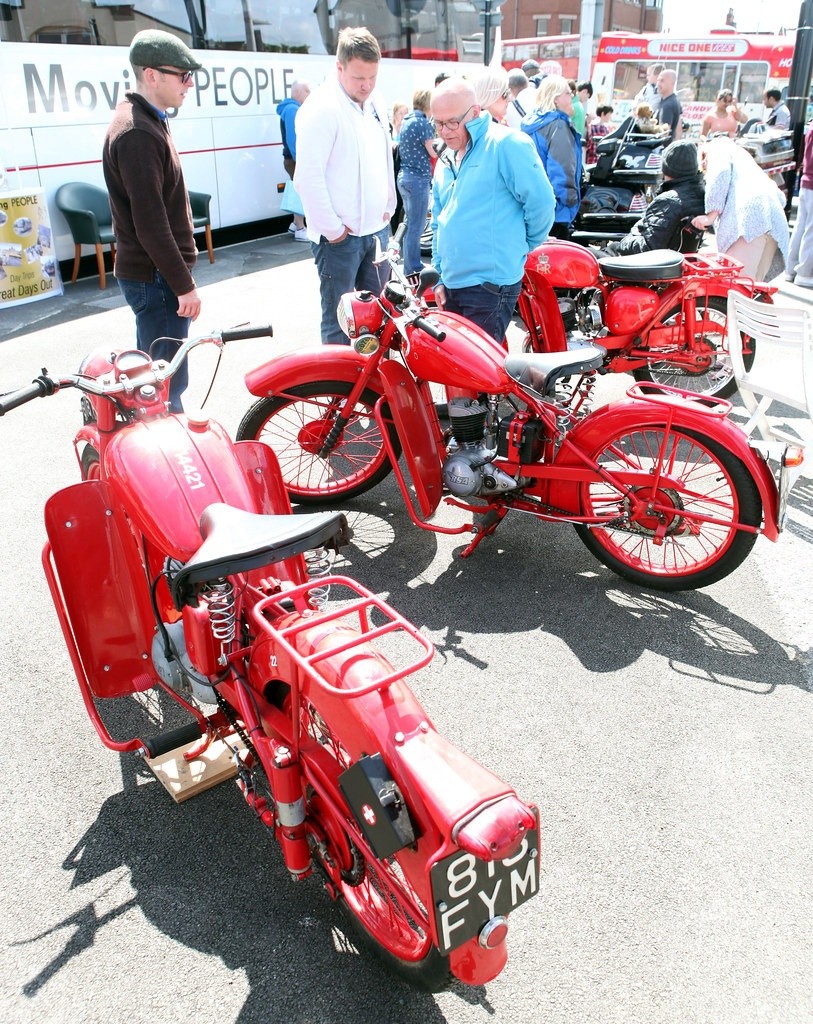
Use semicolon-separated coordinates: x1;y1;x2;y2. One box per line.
724;99;732;103
142;65;193;85
502;87;511;99
428;105;474;132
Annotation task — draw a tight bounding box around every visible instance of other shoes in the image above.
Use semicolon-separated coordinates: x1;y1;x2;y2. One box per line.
784;274;794;281
793;273;813;287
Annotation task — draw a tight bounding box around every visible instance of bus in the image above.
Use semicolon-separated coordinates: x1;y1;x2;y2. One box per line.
0;1;509;270
500;33;596;88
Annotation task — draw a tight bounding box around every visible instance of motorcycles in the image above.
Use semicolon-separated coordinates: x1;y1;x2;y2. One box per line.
237;217;801;590
4;316;542;996
412;237;778;409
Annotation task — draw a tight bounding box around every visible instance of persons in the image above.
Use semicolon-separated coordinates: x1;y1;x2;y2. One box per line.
278;58;813;339
429;77;555;399
292;27;398;419
102;30;202;410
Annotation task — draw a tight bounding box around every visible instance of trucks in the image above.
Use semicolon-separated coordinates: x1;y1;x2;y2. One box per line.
589;32;813;167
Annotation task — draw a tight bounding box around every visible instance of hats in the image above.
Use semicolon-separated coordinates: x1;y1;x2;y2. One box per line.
129;29;202;69
662;140;699;179
522;59;540;72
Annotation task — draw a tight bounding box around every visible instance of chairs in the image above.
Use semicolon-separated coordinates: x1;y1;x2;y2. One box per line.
54;180;117;290
188;190;215;265
725;289;813;495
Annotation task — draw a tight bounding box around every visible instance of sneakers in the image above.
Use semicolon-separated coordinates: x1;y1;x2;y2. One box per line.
288;221;310;242
